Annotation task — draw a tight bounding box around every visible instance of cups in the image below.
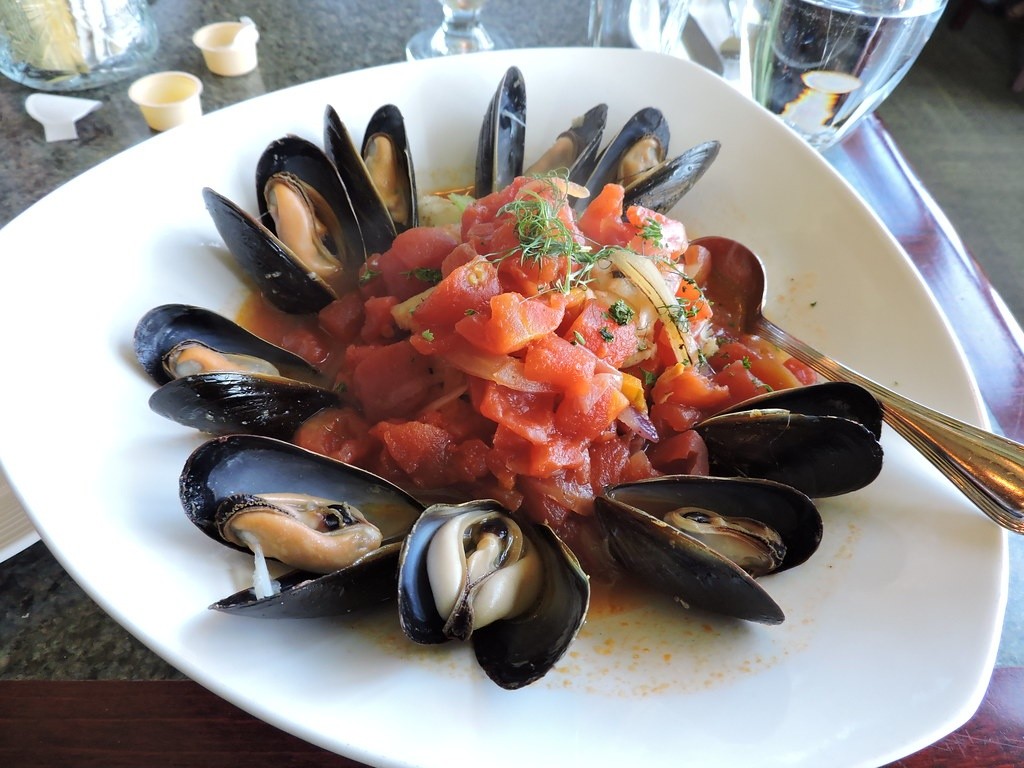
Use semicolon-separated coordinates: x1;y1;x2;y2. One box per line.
0;0;160;91
754;0;948;154
589;0;690;54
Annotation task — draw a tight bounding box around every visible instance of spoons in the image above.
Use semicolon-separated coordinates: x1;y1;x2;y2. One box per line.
682;239;1024;537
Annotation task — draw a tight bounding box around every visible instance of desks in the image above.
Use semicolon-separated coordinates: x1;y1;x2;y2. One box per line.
0;0;1024;768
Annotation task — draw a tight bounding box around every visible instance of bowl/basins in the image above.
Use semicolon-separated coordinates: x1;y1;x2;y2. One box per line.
192;21;258;76
128;70;203;130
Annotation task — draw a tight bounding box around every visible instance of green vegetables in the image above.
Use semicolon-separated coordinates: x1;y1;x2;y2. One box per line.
356;167;777;394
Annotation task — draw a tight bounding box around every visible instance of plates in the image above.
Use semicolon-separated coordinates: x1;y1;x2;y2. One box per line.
0;47;1009;768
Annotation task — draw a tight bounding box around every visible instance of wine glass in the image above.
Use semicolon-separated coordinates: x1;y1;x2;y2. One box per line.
406;0;513;60
